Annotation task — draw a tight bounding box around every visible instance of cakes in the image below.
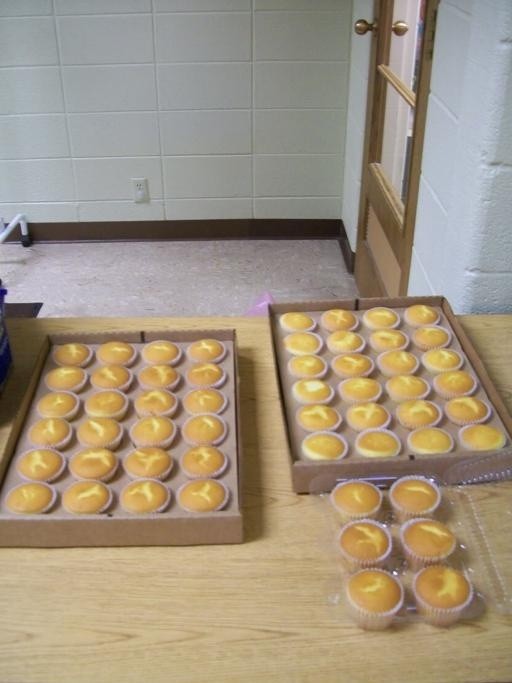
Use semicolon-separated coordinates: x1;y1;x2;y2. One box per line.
397;400;441;429
138;366;179;390
321;309;358;332
446;397;491;426
84;390;129;420
331;352;375;378
386;375;430;402
412;326;450;351
339;378;381;404
414;566;474;627
28;419;72;449
404;305;440;329
188;339;226;364
97;341;136;368
69;449;119;481
76;419;122;448
124;448;173;480
347;403;391;432
130;416;175;448
45;366;87;392
185;362;226;388
364;307;400;330
376;352;419;377
135;390;177;418
424;349;463;373
326;330;365;355
183;413;226;447
292;379;335;405
336;520;392;572
346;568;404;630
370;331;409;353
121;480;170;513
182;388;226;416
180;447;228;480
400;517;457;569
54;342;92;367
7;483;56;514
304;432;345;460
331;480;382;526
433;370;477;401
357;431;399;459
63;479;112;514
38;391;80;420
18;448;64;483
410;428;453;457
142;341;181;367
177;479;229;513
390;475;441;522
284;333;323;355
460;425;505;454
281;313;316;333
288;355;327;380
91;366;133;391
296;405;342;433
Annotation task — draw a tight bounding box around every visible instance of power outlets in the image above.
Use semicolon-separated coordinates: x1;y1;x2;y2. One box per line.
132;179;150;203
